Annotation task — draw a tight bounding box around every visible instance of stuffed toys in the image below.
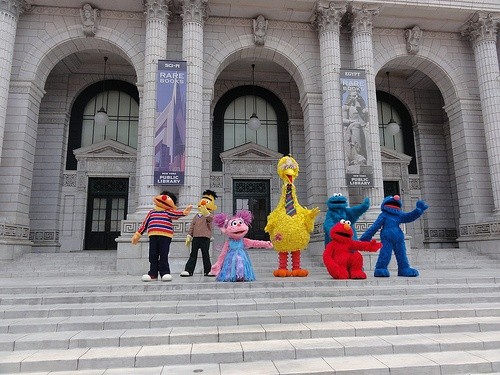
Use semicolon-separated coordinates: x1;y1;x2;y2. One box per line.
323;219;382;279
359;195;429;277
131;190;194;281
211;210;274;282
179;189;217;276
323;193;370;271
264;155;320;277
